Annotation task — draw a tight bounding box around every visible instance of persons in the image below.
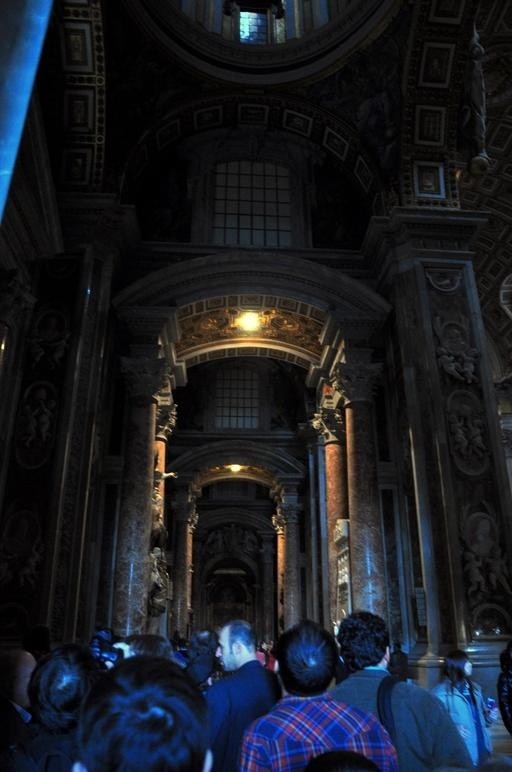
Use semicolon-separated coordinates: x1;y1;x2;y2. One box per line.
237;608;477;772
428;649;499;769
477;755;512;772
0;618;283;772
496;640;512;734
